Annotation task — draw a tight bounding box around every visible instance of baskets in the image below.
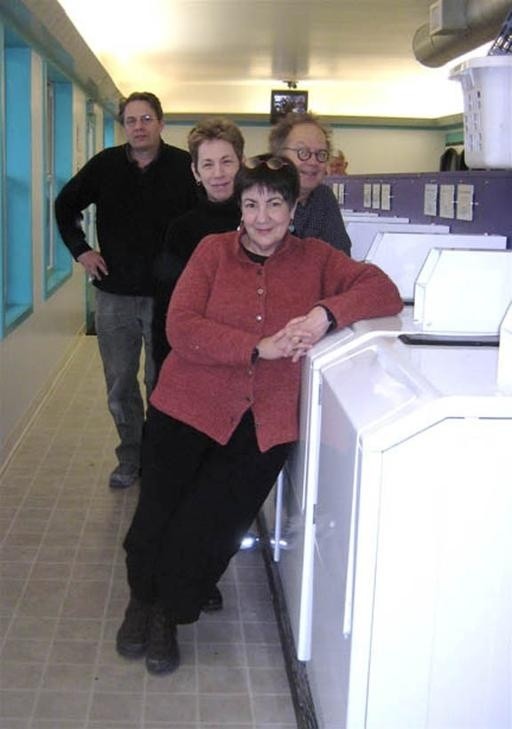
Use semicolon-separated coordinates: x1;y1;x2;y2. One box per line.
448;54;512;170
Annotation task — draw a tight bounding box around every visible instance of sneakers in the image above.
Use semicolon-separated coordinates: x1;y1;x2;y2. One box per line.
143;608;179;677
109;464;139;488
202;587;222;612
116;609;147;658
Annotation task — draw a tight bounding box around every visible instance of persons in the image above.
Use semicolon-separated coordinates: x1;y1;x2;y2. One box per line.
53;92;190;489
259;111;350;259
151;116;254;615
109;155;403;677
327;149;349;176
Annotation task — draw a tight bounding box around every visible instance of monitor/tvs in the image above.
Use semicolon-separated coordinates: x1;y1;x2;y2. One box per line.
271;90;308;126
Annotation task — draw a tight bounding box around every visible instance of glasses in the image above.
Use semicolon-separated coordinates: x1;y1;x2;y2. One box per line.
244;157;289;171
126;115;160;126
281;146;330;163
